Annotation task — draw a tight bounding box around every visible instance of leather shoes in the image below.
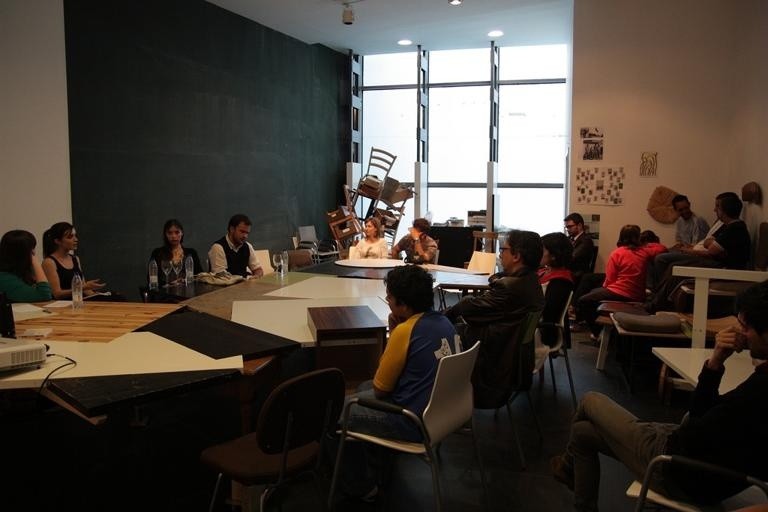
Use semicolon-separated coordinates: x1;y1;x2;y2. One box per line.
550;455;574;492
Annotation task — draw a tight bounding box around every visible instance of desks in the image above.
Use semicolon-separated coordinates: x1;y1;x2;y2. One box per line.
1;298;301;482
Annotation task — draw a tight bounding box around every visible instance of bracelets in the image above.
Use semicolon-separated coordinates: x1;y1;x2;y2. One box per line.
414;238;420;244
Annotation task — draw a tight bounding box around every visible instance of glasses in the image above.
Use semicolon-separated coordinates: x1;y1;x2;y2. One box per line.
566;224;576;229
499;247;510;253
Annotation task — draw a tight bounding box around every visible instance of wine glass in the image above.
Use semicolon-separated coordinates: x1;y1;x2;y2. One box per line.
160;259;173;288
272;254;283;276
173;258;184;287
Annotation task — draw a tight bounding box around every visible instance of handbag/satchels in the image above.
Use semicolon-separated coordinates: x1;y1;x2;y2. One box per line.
198;272;243;286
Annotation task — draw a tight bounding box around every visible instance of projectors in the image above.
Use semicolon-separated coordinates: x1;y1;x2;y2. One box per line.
0;336;47;372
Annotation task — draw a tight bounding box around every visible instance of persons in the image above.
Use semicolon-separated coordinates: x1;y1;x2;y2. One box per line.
40;221;127;302
355;216;389;258
584;142;600;159
546;282;768;511
207;214;263;281
392;217;438;265
332;264;464;501
147;218;203;286
0;229;53;304
442;229;544;407
535;180;767;362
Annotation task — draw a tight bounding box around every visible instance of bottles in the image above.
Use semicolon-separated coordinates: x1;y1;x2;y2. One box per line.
185;252;194;283
149;255;158;290
71;268;83;312
282;249;288;273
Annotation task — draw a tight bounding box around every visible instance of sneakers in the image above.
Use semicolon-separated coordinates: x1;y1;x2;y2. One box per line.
570;325;585;331
